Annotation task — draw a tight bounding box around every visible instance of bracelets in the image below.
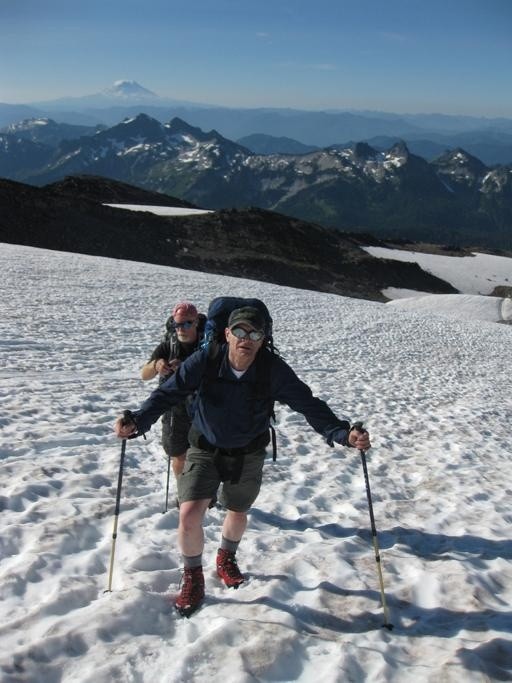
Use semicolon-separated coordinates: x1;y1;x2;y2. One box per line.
154;358;158;372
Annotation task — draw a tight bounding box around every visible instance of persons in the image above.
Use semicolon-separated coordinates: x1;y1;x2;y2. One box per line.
112;305;373;616
140;302;208;508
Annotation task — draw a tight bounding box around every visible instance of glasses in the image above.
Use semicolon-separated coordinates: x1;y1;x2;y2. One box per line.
172;319;197;329
230;327;265;342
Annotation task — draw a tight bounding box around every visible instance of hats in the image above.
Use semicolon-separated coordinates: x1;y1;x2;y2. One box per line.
228;306;266;331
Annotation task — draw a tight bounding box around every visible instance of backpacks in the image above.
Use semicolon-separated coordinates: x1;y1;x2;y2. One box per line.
200;296;273;456
161;312;207;363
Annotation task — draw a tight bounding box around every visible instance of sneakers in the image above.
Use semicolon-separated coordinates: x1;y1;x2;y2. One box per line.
174;585;205;614
216;562;245;588
